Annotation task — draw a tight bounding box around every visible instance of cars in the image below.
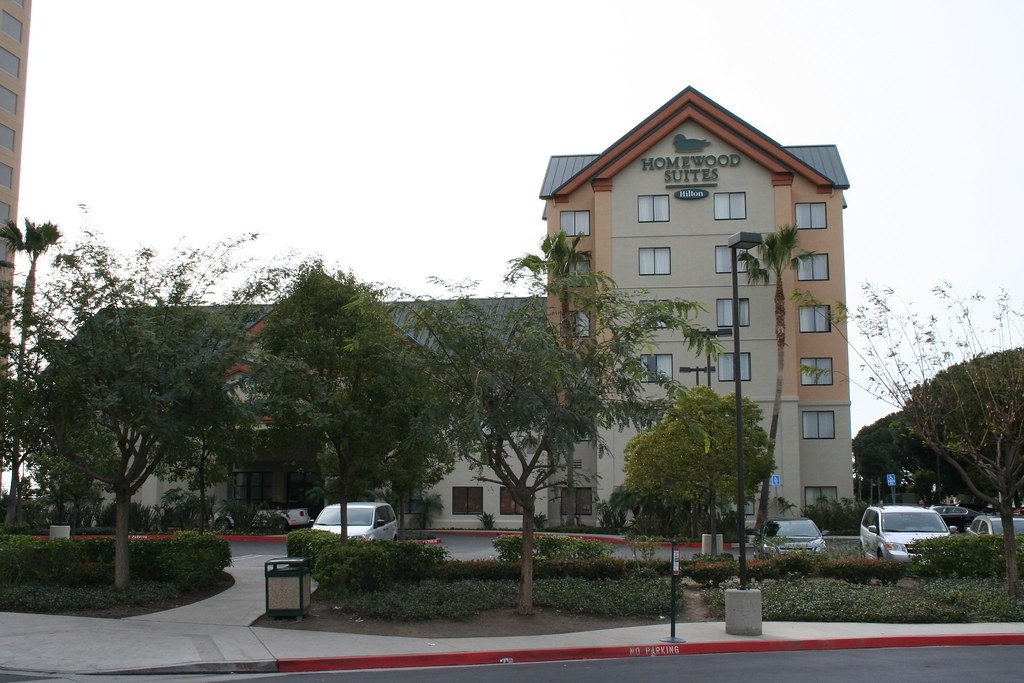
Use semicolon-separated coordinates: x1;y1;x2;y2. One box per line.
207;497;310;535
750;517;832;560
965;514;1024;537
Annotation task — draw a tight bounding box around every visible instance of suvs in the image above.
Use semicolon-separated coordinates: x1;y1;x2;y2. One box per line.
309;502;400;542
859;501;958;564
929;504;986;533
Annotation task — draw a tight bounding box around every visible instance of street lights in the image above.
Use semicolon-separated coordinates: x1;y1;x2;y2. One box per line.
728;230;764;592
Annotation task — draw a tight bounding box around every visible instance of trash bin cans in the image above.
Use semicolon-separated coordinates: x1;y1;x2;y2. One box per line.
264;557;314;621
